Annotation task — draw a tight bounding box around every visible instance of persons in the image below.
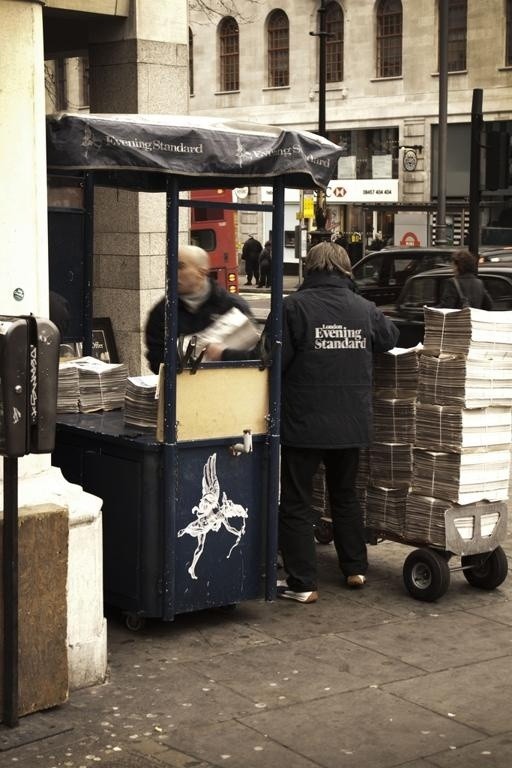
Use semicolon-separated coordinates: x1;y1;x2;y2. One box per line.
260;240;402;604
439;250;494;310
254;242;273;287
241;232;264;285
141;242;264;378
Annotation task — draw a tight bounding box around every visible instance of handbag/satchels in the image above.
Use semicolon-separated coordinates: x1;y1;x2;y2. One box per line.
177;307;261;363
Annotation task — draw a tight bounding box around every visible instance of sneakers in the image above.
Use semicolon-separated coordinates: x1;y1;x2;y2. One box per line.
277;580;318;602
244;281;265;288
347;575;368;588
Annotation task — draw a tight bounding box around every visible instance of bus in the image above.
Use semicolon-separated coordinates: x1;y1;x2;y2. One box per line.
190;188;239;298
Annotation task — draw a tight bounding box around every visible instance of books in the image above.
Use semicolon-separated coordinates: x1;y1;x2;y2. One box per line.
182;307;259;360
53;352;161;431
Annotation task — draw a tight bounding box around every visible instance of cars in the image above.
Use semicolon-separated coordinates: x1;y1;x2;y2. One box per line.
350;248;512;348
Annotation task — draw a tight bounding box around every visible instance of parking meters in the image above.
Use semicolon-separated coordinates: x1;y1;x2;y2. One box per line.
0;312;60;726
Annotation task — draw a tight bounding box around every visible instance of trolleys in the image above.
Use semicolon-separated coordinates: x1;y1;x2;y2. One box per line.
47;112;342;631
312;511;509;602
